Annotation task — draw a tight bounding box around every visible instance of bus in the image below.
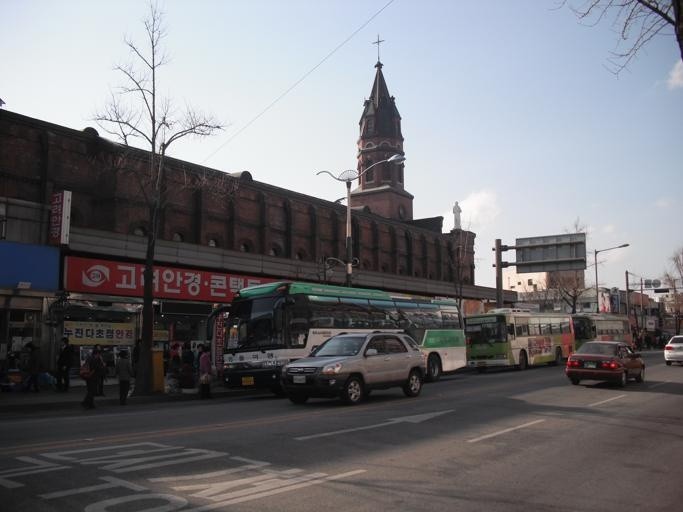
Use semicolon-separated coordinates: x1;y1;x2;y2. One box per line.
463;308;576;374
570;313;633;351
205;280;468;397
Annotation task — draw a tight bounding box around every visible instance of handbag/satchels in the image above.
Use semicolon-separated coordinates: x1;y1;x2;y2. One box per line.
79;362;95;379
198;374;213;385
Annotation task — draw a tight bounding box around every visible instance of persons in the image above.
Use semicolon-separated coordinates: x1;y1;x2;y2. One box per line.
80;348;108;408
115;350;136;405
20;341;43;392
53;336;74;392
452;201;462;230
634;333;670;351
164;336;215;400
91;344;107;396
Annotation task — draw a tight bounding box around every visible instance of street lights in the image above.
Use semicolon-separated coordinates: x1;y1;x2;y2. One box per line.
594;243;629;313
316;153;407;287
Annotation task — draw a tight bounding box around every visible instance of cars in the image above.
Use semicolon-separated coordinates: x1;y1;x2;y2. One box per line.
279;330;427;404
565;340;645;388
663;334;682;366
